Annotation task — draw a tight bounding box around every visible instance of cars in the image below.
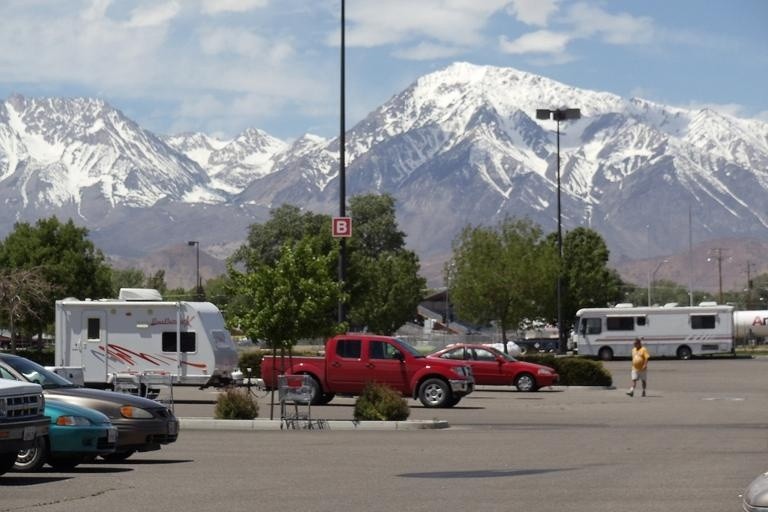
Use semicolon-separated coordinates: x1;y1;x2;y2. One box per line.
425;343;561;392
0;351;181;477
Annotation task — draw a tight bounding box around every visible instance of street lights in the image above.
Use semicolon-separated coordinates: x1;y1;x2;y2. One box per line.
536;104;582;357
187;239;200;293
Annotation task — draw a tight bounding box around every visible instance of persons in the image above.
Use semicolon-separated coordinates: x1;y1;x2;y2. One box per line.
625;337;650;398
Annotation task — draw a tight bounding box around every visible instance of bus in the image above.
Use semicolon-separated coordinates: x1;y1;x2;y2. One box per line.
568;301;736;361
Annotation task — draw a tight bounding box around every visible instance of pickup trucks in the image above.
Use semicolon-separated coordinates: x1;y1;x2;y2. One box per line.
261;334;476;409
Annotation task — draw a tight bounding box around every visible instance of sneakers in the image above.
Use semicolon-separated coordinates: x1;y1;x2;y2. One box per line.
627;392;632;396
642;391;645;396
